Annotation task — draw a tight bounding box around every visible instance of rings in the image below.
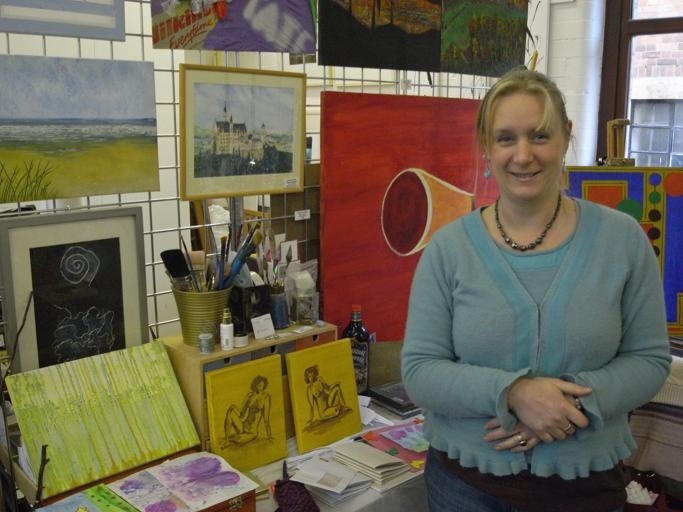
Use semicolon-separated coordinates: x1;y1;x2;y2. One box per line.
515;432;528;446
563;424;573;433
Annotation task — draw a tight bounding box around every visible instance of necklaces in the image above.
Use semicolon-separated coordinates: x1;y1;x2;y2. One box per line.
494;187;562;254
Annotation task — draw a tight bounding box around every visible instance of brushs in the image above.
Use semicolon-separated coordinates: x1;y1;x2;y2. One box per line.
160;250;207;292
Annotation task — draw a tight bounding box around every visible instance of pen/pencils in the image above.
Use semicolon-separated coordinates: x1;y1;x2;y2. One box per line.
264;276;285;295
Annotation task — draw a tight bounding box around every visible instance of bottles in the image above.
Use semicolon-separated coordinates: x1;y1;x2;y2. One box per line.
218;306;249;350
341;305;371;394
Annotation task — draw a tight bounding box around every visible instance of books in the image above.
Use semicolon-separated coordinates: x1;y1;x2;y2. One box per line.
251;379;430;507
620;350;683;485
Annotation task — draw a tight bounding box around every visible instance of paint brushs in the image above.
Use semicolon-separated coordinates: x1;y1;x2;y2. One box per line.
180;222;293;292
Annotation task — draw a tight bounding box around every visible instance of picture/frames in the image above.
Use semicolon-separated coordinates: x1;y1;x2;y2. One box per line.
1;205;148;376
178;63;308;204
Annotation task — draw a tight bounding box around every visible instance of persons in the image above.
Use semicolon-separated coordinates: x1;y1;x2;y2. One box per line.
399;66;672;512
218;375;275;449
302;364;352;424
